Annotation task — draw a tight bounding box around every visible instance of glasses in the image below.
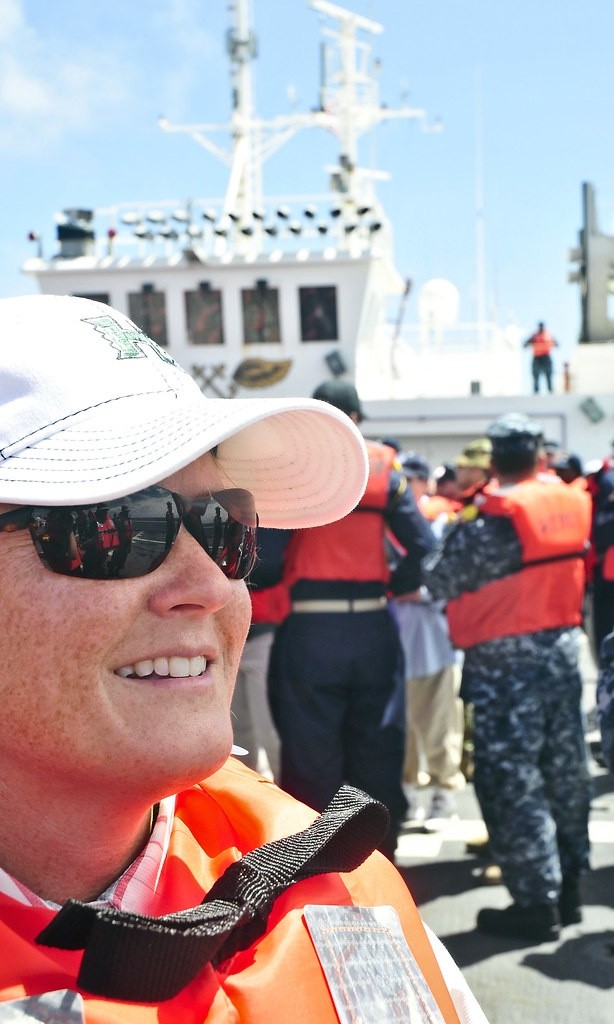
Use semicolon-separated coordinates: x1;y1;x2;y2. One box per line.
0;486;259;585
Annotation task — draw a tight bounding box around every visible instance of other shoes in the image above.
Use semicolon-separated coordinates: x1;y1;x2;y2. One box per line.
402;787;461;830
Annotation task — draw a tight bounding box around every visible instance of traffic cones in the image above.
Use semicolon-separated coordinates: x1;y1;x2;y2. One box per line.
67;531;84;571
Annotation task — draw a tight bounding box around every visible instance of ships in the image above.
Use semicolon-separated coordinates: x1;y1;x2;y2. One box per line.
16;2;614;477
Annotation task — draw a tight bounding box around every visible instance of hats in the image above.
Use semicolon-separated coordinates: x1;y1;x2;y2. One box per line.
455;438;494;468
167;501;172;507
400;456;428;481
122;506;132;511
96;502;109;510
0;294;372;530
311;379;372;422
483;407;545;454
215;506;221;510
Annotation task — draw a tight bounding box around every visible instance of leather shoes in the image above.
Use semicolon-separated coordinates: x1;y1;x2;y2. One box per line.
476;896;581;942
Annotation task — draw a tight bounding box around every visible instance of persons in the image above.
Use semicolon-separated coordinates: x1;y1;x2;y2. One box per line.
0;294;493;1024
228;528;290;785
398;411;591;940
44;500;173;577
313;366;366;424
368;435;614;833
212;503;256;579
245;377;441;861
522;322;558;394
